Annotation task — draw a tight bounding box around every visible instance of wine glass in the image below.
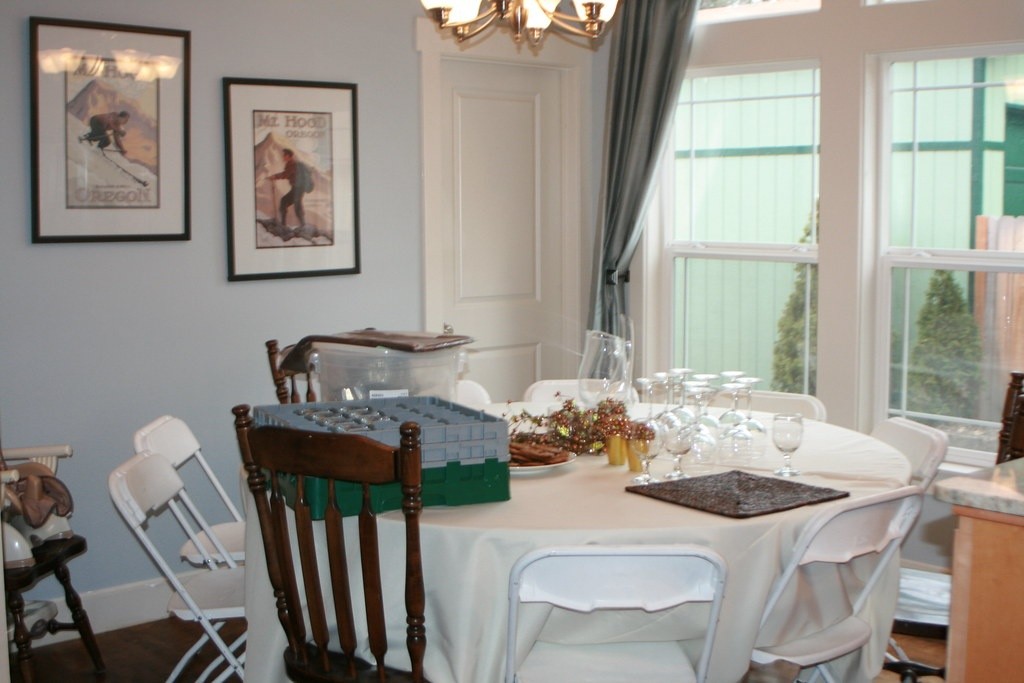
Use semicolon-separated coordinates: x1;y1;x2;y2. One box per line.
629;367;767;482
772;413;804;477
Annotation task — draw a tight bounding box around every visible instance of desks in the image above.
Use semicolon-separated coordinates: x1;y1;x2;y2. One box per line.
932;457;1024;683
238;399;915;683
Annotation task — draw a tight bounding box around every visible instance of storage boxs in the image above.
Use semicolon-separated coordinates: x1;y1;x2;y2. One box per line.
253;396;510;469
259;459;511;522
310;335;473;404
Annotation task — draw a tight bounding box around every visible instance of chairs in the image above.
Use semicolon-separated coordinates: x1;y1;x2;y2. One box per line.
748;485;927;683
713;389;827;423
0;441;104;683
523;379;640;404
414;380;492;405
231;402;431;683
504;543;729;683
105;450;249;683
871;416;951;664
264;326;374;405
133;416;246;570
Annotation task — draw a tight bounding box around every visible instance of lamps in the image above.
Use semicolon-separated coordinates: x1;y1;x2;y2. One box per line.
39;30;184;83
421;0;617;47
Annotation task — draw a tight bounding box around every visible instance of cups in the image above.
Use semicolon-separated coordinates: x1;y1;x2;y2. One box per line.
577;329;633;405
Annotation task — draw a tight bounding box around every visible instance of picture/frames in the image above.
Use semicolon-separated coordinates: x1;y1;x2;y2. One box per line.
28;15;190;243
221;77;363;281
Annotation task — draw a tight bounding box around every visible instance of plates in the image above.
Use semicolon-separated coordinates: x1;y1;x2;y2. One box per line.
509;452;577;478
